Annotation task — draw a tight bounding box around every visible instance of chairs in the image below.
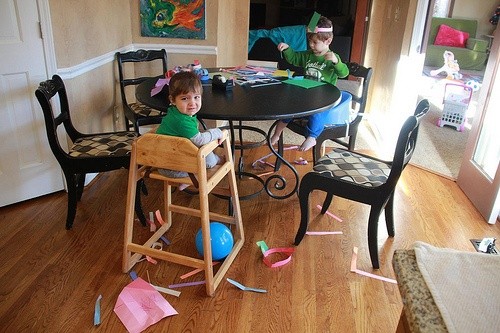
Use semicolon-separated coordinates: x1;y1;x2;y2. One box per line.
116;49;168;133
274;62;373;172
292;98;430;269
122;129;245;296
34;74;147;230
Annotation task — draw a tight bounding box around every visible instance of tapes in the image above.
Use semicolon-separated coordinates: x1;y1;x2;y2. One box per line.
219;76;227;83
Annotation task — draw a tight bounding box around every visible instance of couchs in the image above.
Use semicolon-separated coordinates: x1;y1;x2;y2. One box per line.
424;17;489;70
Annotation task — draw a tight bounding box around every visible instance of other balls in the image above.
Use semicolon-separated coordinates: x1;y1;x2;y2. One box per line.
195;222;234;260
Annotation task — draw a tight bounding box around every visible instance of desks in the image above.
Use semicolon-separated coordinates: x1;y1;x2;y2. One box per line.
135;67;342;228
392;240;500;333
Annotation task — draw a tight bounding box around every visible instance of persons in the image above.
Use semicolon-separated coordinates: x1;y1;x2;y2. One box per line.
431;51;463;81
150;72;225;191
271;15;350;152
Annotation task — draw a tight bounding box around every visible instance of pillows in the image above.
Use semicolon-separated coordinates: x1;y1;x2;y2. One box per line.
433;24;469;48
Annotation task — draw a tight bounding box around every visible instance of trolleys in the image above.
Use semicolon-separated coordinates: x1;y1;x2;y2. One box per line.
436;82;474;133
